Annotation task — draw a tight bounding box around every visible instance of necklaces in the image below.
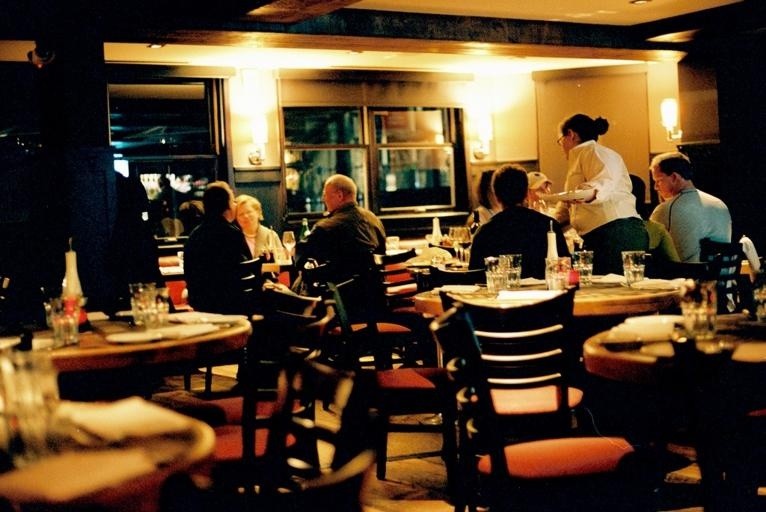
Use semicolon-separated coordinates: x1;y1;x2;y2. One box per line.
243;231;255;238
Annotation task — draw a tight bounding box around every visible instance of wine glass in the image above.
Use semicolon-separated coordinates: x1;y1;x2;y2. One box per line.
283;232;296;265
448;226;472;268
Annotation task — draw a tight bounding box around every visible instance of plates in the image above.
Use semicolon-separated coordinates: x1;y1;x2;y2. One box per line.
426;233;472;271
626;312;766;366
106;310;248;344
536;184;598;202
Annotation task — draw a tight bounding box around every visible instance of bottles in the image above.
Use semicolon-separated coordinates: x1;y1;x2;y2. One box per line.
60;251;92;331
357;189;364;209
433;217;451;247
546;219;558;290
1;348;63;473
469;210;482;237
300;218;311;243
683;282;716;340
305;193;325;213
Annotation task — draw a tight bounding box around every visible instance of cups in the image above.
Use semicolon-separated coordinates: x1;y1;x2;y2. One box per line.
546;250;645;291
49;297;80;348
484;254;521;294
385;236;400;253
129;282;170;333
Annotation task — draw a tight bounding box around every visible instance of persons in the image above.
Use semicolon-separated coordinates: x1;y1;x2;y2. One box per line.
183;180;281;316
154;176;185;203
468;152;733;361
234;192;287;291
293;174;388;321
550;113;651;283
